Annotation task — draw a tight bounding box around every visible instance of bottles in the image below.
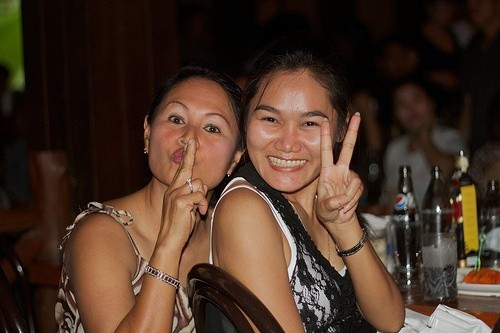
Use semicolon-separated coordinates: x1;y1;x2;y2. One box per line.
421;164;452;210
392;164;420;215
479;180;500;268
450;148;479;268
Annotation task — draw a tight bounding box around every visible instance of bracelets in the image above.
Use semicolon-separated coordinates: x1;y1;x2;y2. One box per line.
335;227;368;256
144;265;181;289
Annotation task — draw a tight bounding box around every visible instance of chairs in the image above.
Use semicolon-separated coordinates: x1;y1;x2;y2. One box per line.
0;250;39;333
187;263;285;333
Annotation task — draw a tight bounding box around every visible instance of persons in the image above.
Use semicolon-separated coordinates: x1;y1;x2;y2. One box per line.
55;65;246;333
235;0;500;216
0;62;40;209
210;49;406;333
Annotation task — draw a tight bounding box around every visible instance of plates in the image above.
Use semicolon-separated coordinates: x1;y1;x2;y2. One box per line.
456;268;500;293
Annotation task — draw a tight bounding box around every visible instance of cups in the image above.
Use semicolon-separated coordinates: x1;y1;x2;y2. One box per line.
384;213;425;305
415;207;460;304
